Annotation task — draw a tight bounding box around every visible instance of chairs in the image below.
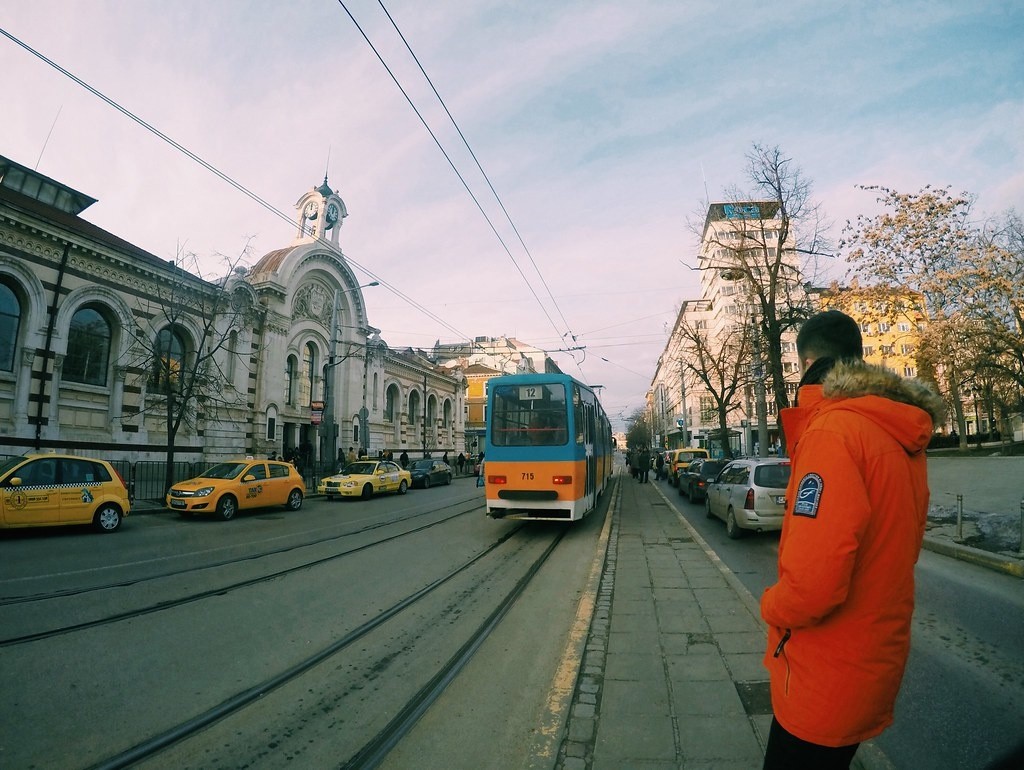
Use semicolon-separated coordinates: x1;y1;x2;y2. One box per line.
768;470;783;483
39;463;53;485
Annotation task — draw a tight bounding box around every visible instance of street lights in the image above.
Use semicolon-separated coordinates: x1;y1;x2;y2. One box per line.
966;420;975;436
971;386;983;451
719;265;771;463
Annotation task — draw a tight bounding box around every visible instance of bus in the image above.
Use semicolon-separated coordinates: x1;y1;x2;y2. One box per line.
480;373;617;524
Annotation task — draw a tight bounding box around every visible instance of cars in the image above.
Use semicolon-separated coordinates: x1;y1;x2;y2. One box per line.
0;454;132;534
675;457;732;503
404;460;453;489
166;456;306;521
704;455;793;538
474;463;481;477
649;447;673;479
317;460;413;502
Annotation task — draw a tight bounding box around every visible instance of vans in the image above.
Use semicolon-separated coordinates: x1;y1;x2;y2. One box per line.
666;448;709;488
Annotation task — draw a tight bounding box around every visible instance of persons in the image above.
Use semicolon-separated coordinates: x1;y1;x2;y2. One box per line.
382;450;393;461
443;452;450;465
466;451;470;459
761;310;951;770
338;448;345;462
269;447;306;480
520;426;532;444
358;448;367;460
777;437;782;457
476;452;485;488
950;430;957;444
348;447;357;462
654;451;665;480
400;450;409;467
424;452;431;459
458;453;466;472
626;447;650;483
307;439;314;467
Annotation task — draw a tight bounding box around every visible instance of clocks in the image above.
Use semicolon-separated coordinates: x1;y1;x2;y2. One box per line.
328;204;338;221
304;201;318;217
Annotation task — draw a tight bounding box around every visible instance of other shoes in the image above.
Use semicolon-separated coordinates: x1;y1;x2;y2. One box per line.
636;479;638;481
638;481;643;483
654;479;659;481
633;479;635;481
644;482;648;483
